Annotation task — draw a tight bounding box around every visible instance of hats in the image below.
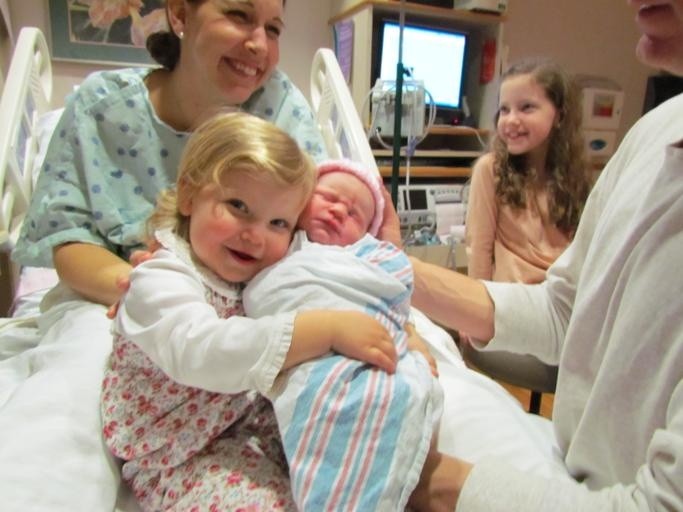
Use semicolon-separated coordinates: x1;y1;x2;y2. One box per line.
317;159;385;237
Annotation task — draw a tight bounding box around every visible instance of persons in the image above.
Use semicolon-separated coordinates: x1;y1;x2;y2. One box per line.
98;107;443;510
462;53;588;286
389;1;683;509
245;158;442;511
4;3;330;510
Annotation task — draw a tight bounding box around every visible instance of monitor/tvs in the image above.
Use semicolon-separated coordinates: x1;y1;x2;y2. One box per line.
371;18;468;116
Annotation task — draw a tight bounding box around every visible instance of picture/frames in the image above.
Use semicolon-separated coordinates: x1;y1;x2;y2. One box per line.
47;0;173;70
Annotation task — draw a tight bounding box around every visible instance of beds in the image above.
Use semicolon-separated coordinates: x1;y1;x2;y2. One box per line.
0;25;557;511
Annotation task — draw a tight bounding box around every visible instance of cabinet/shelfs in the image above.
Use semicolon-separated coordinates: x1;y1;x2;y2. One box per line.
328;0;508;178
